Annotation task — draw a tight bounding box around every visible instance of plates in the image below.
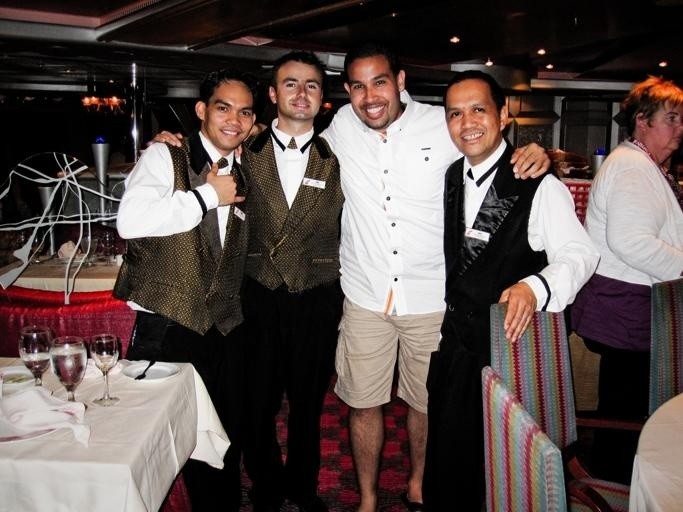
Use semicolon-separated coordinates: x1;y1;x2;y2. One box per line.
121;361;181;383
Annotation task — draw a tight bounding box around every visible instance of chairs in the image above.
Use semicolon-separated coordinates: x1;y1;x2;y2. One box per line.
481;302;628;512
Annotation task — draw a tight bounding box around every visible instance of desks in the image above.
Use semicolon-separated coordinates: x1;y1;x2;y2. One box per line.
626;393;683;509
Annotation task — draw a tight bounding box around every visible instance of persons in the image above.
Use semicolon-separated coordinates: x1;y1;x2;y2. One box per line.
320;41;551;510
421;68;600;511
146;51;345;510
569;74;683;486
114;73;254;512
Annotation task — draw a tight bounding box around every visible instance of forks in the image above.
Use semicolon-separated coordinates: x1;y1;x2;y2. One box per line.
134;360;156;381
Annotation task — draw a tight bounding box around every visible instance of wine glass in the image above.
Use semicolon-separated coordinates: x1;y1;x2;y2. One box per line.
28;230;46;265
79;232;115;269
19;324;121;409
